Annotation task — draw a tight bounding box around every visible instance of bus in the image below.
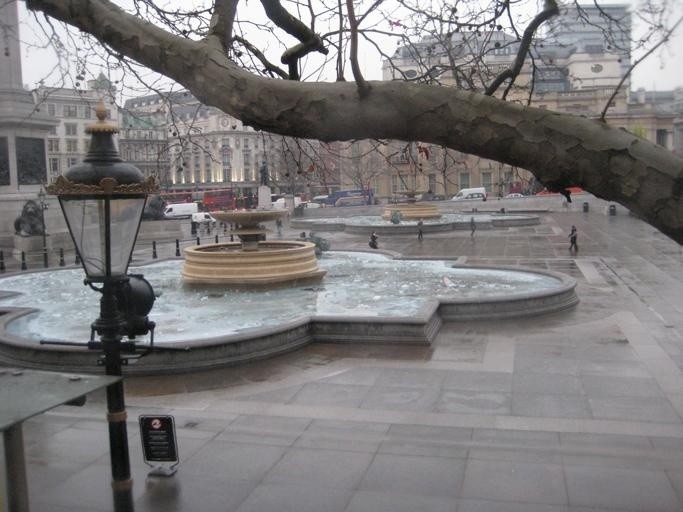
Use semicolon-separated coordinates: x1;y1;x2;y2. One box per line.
160;190;236;213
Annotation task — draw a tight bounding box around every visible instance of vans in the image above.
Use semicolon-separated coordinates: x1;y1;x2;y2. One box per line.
450;187;486;200
162;202;199;220
190;212;217;224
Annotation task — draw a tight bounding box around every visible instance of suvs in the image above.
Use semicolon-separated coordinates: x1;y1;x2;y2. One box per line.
459;193;485;200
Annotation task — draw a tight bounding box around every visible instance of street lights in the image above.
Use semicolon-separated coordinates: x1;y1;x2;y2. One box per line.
38;188;50;267
41;96;161;512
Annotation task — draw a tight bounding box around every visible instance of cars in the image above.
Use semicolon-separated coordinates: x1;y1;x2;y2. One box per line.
271;188;374;209
506;193;524;198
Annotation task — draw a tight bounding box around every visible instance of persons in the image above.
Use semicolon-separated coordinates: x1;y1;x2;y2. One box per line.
369;230;379;249
260;161;268;186
470;217;477;236
276;218;283;234
568;226;578;251
417;217;424;240
